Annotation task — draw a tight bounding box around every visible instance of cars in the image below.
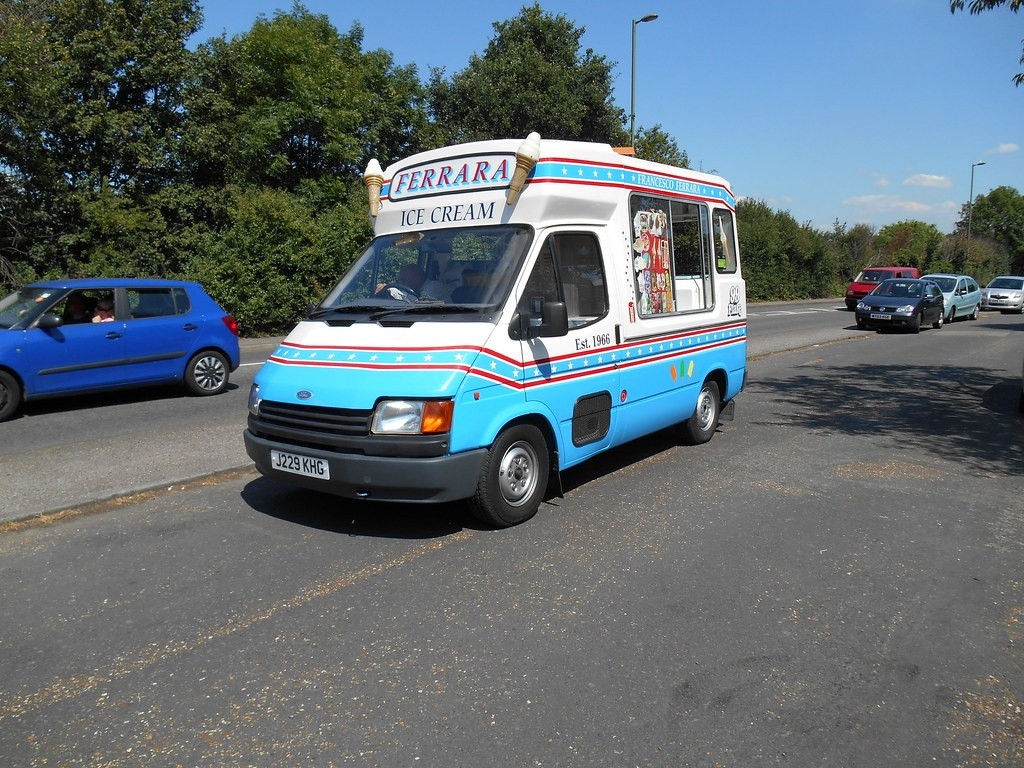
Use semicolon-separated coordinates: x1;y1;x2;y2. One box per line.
919;273;982;323
854;278;946;334
981;276;1024;314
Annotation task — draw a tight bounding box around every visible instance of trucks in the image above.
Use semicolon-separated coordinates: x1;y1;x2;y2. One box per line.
241;131;748;530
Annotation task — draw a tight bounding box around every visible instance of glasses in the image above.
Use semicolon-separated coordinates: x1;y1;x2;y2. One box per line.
69;300;80;304
95;305;109;312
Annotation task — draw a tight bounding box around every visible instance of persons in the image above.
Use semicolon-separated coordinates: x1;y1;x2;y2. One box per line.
376;263;453;306
91;299;114;323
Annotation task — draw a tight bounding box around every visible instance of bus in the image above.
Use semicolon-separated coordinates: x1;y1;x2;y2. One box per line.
845;267;920;310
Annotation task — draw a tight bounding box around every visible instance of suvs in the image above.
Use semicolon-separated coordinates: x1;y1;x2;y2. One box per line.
0;277;242;424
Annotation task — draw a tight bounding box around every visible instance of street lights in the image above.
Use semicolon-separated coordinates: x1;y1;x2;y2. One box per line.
966;161;987;253
629;12;660;150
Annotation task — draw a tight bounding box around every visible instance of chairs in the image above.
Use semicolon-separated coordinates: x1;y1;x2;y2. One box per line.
895;287;908;297
63;304;113;322
